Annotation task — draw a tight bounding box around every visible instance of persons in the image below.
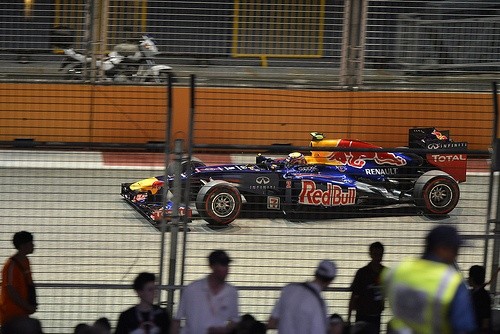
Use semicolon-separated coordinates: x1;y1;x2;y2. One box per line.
172;251;239;334
0;230;38;328
348;239;393;334
467;265;494;334
0;319;44;334
378;226;473;334
261;259;338;334
114;273;171;334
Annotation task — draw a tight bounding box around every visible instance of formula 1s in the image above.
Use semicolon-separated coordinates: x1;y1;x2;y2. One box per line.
119;125;469;233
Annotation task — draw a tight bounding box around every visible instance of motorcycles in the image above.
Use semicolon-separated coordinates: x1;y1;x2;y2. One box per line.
57;34;175;86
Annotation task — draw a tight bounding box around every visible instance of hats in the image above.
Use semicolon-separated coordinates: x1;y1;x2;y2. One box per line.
425;226;472;248
316;259;337;278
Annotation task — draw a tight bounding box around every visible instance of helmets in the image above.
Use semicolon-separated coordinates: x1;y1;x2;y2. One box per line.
310;130;324;141
289;152;304;161
